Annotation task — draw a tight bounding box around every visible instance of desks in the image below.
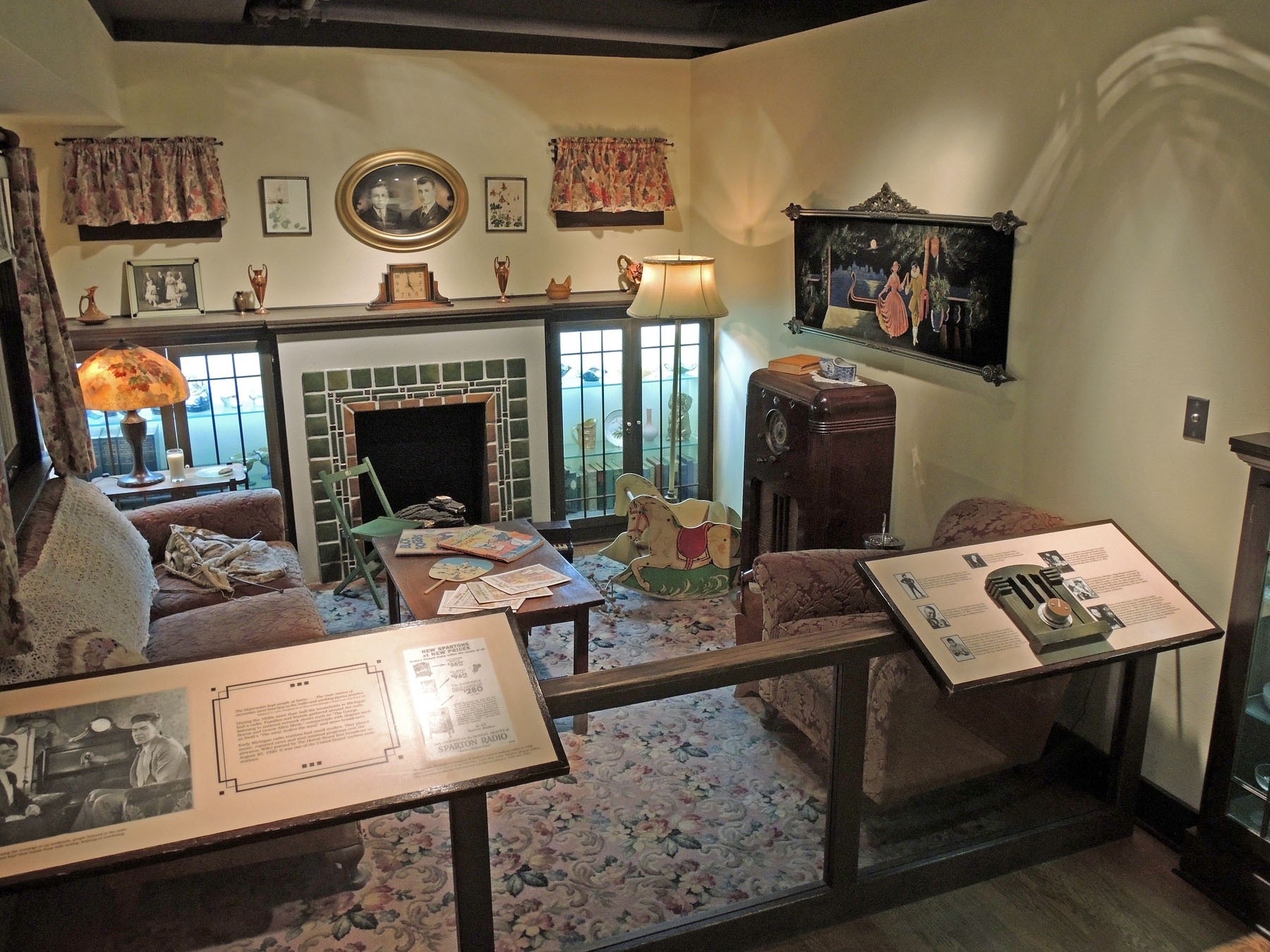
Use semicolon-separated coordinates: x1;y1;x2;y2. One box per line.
371;519;605;733
91;463;247;500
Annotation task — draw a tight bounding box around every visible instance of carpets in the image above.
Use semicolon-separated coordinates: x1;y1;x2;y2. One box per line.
0;556;1009;952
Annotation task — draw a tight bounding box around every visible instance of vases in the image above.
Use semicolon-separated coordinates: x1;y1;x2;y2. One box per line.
235;264;270;315
643;408;658;442
75;285;112;324
494;255;511;303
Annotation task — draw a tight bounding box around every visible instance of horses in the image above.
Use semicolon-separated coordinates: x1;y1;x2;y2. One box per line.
612;492;741;591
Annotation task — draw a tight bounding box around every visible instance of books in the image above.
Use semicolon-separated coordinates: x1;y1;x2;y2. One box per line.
767;354;824;375
395;523;543;564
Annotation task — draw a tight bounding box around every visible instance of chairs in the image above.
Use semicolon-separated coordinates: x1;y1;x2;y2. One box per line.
319;457;424;610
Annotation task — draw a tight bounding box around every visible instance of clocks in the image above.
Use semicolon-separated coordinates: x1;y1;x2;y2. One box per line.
390;262;429;303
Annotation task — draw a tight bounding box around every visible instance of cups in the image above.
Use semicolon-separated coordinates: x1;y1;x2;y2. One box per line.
166;449;186;483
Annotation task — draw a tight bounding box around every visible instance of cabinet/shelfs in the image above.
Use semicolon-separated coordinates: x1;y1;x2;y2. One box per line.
546;307;713;545
1173;431;1270;939
72;338;272;507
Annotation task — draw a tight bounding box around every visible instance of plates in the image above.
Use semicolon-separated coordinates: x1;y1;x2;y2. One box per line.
196;466;233;477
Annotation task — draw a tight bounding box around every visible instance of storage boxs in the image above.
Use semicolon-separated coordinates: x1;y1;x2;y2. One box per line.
89;423;159;479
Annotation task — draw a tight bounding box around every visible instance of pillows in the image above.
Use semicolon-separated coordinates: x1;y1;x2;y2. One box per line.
55;627;151;677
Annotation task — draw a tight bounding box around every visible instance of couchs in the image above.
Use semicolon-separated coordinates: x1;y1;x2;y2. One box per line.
0;476;326;686
751;497;1079;847
27;744;193;842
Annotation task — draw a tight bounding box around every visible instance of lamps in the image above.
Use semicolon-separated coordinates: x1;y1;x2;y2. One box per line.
626;249;728;503
76;336;191;488
14;711;63;796
765;409;789;456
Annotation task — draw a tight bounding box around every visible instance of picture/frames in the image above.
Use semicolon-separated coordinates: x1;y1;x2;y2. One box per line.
125;257;206;319
261;148;527;254
779;182;1028;388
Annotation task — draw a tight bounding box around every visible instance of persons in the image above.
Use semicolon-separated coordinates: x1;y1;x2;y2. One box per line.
900;574;969;656
0;712;189;847
1046;552;1114;629
143;270;188;309
969;554;984;569
361;174;448;234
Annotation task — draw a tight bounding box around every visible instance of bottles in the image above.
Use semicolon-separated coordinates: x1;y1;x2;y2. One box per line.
76;286;112;324
233;291;247;315
